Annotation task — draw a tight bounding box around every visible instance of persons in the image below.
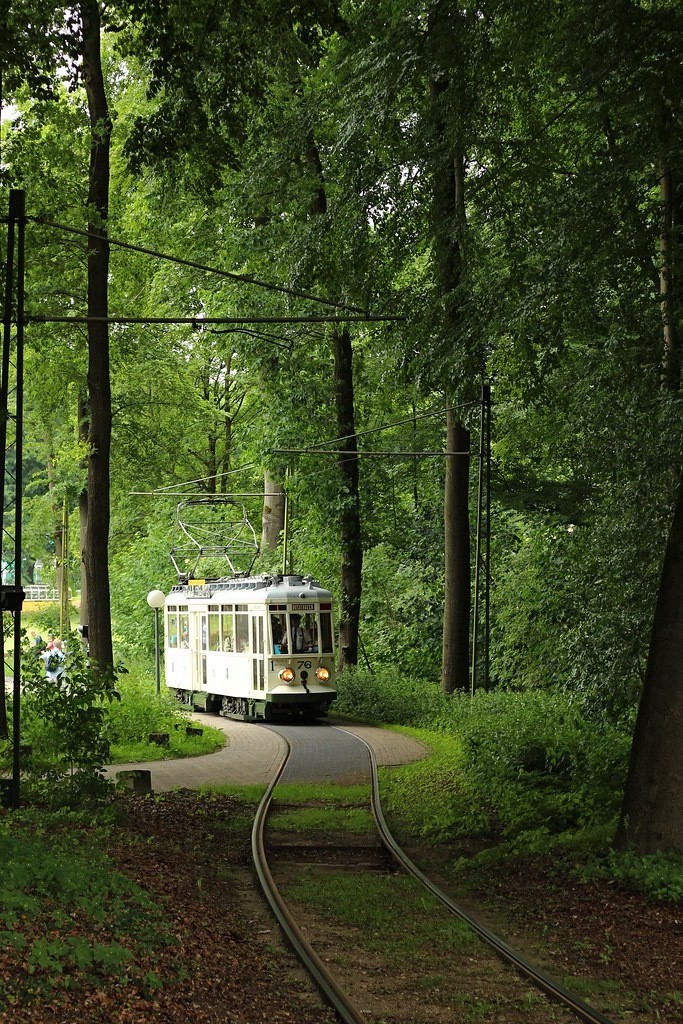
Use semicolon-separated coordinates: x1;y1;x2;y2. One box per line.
209;614;249;653
31;631;46;658
271;613;326;654
169;614;189;649
46;634;61;654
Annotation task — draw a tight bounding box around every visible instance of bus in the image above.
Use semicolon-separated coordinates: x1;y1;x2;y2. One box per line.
162;572;339;723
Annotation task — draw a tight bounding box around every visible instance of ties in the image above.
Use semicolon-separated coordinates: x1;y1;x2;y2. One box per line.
293;631;297;651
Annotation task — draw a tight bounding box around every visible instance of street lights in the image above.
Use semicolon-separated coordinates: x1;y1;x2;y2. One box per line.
148;590;166;695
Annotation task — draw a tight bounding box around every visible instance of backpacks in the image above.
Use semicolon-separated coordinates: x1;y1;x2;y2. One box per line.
45;652;60;672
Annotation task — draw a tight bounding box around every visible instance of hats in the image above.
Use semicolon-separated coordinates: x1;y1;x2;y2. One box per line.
292;614;302;620
53;638;60;646
